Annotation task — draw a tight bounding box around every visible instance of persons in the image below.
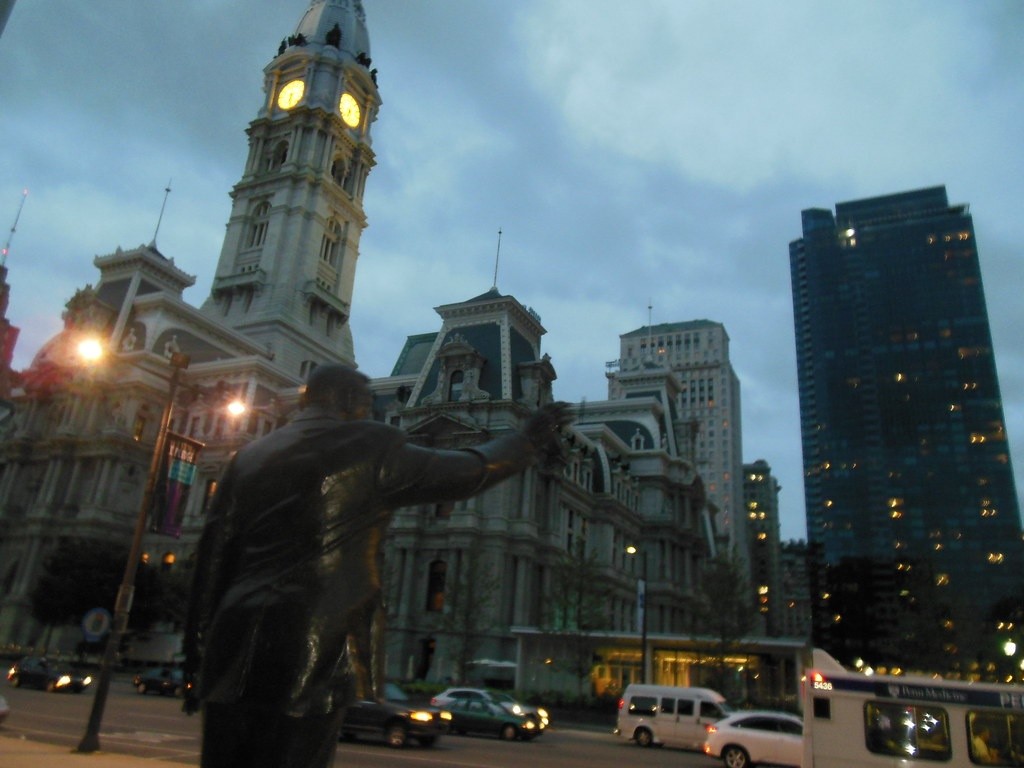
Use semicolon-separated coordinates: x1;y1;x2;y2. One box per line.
182;364;574;768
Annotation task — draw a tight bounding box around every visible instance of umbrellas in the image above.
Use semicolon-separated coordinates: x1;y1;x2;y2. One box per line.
490;661;516;684
465;658;499;684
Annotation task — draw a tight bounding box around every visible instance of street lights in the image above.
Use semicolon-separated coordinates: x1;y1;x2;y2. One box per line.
1004;640;1016;685
626;547;647;684
76;336;248;751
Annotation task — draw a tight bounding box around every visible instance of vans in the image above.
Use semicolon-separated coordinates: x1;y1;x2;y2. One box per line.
614;684;734;754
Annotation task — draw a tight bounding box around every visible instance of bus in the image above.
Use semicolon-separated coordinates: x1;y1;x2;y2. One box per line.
801;648;1023;768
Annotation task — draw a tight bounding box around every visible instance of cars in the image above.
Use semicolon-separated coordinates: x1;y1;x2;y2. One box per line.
134;665;190;698
704;712;803;768
7;655;91;695
334;676;452;749
427;686;548;741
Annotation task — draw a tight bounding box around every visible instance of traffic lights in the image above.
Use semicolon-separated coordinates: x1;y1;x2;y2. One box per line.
137;553;148;578
161;550;175;573
